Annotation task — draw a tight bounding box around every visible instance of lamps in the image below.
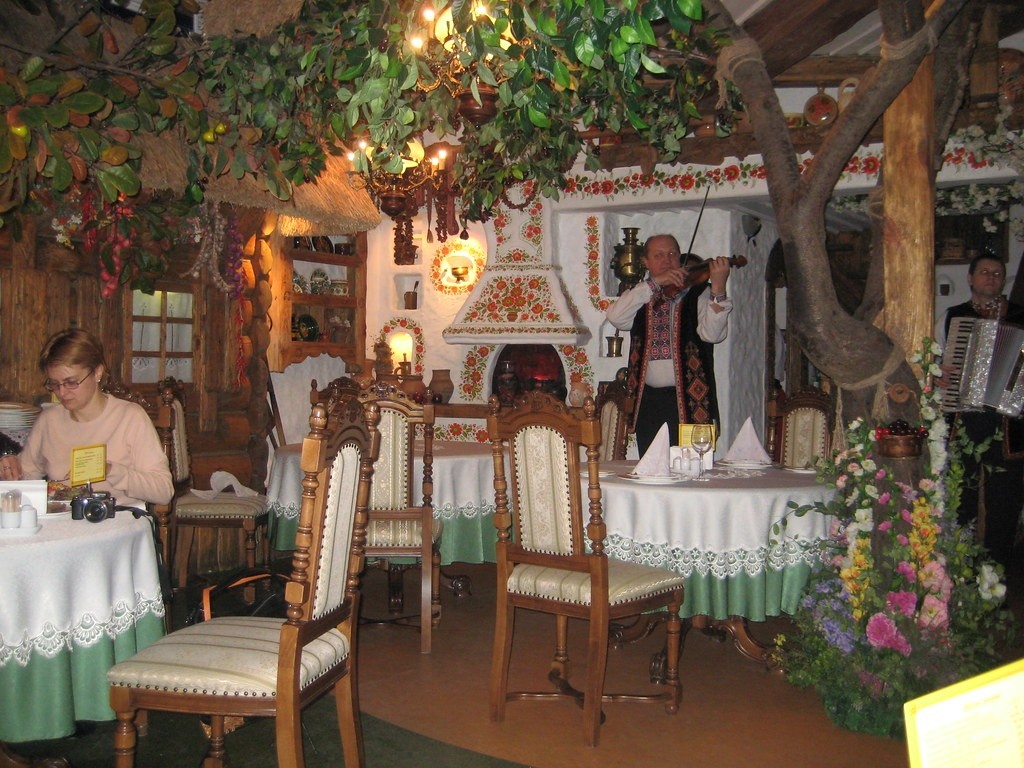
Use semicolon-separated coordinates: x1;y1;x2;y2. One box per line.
349;137;447;266
409;2;520;130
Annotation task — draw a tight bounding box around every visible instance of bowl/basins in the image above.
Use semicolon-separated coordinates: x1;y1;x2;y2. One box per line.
878;434;922;457
451;266;468;282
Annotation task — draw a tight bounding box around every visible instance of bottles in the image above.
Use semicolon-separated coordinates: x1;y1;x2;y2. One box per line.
428;369;455;405
401;375;426;398
20;504;37;528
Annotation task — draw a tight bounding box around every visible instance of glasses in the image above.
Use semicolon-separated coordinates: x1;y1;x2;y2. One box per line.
980;270;1000;277
43;369;94;391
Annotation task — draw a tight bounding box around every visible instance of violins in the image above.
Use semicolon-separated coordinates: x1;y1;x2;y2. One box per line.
666;253;749;296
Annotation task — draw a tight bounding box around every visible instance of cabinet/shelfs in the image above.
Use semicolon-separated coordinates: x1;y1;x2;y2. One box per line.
268;225;366;377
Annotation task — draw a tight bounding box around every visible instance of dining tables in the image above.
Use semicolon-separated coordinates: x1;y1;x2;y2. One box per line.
0;497;169;768
269;434;510;624
566;455;847;690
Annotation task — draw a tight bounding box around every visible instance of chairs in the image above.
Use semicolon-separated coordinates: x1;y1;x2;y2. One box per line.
106;393;380;767
348;378;443;654
485;393;685;750
160;381;272;606
773;385;836;470
101;380;175;629
595;378;633;462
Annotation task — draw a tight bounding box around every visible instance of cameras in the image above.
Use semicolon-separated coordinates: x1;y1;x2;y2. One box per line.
70;491;116;523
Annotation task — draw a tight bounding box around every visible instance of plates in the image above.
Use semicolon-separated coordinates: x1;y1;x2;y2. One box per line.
46;493;106;519
782;468;816;474
579;471;616;478
614;472;692;484
0;524;42;536
714;460;773;470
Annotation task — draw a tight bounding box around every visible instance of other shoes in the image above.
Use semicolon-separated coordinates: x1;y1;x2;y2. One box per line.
65;719;97;742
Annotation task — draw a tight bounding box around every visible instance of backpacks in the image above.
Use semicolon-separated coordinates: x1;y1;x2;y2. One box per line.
170;554;295;632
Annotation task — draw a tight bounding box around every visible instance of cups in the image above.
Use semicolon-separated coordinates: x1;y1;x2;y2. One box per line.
404;291;417;309
0;511;19;528
605;336;624;357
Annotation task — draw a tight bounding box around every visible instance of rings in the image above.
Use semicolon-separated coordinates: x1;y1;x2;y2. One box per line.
4;466;11;469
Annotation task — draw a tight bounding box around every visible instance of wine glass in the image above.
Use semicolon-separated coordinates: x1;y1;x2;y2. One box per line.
691;424;713;482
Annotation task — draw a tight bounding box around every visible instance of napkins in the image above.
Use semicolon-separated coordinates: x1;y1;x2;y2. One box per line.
631;422;672;475
721;416;773;463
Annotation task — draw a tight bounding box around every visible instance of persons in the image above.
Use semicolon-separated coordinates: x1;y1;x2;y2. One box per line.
606;234;734;462
0;326;175;513
934;252;1024;529
